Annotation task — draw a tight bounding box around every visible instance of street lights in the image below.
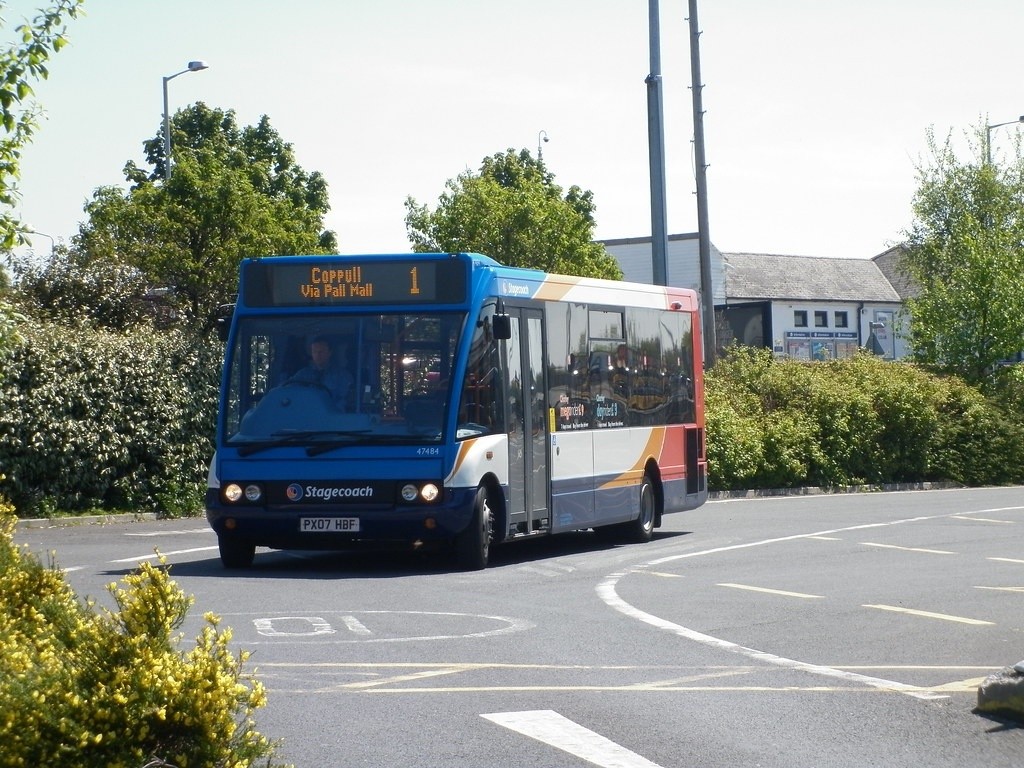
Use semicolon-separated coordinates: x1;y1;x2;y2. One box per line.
985;115;1024;225
162;61;210;319
538;129;550;181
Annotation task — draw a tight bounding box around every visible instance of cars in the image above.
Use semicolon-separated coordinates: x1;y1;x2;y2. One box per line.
399;356;476;388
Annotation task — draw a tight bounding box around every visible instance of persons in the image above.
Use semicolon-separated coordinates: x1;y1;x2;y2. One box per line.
278;339;356;405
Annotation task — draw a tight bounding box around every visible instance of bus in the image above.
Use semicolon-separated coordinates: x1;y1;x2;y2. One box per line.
206;252;708;575
